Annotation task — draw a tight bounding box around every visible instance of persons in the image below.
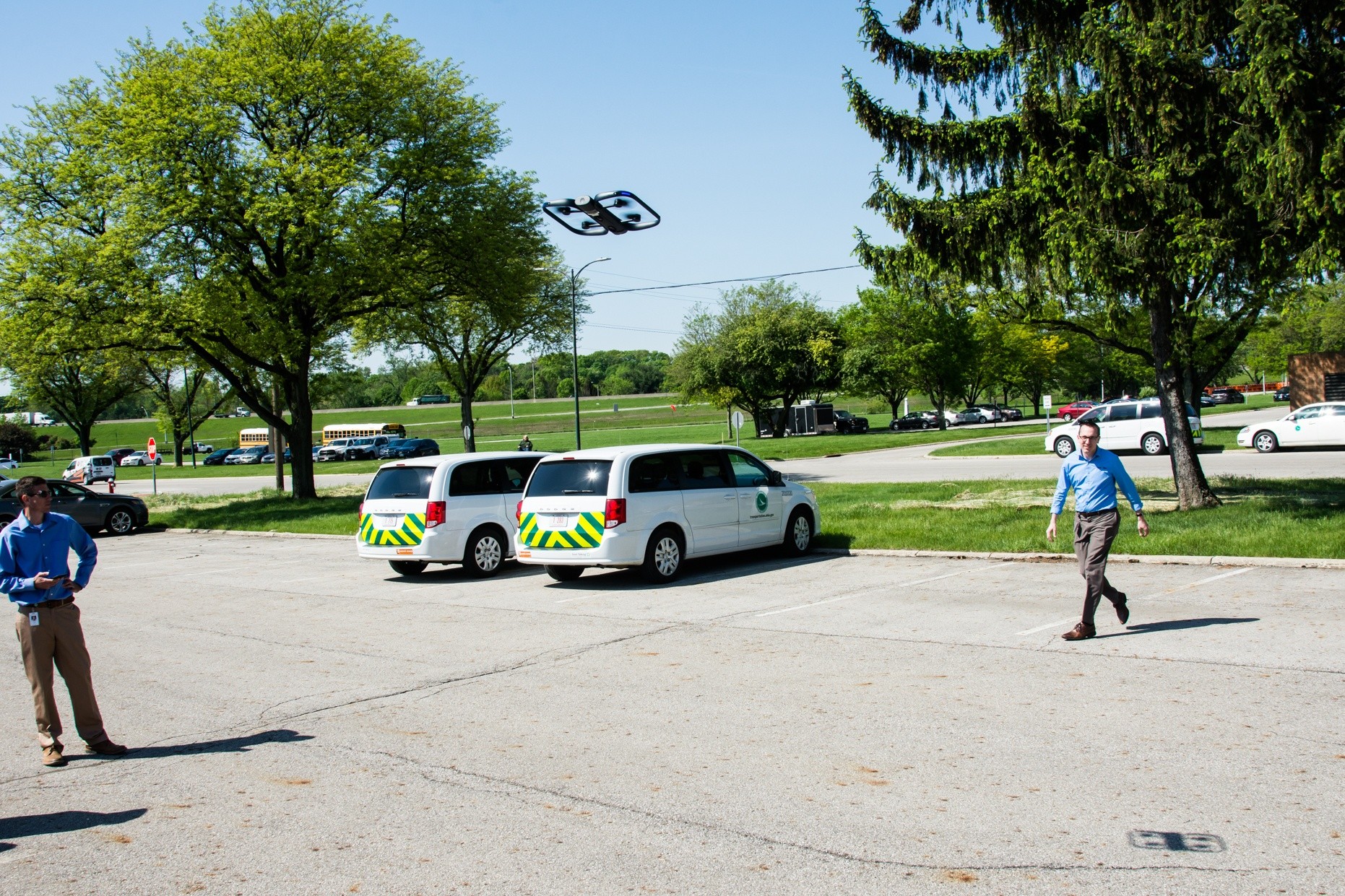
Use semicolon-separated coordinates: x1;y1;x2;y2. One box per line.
1094;409;1104;421
1046;421;1148;641
518;435;532;451
0;476;129;767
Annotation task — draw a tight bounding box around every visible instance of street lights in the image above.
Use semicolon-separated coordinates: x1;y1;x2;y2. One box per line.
508;366;514;419
531;257;613;451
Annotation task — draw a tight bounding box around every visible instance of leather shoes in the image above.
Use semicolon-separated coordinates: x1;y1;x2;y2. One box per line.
1062;621;1096;640
85;739;127;755
42;744;64;766
1113;593;1129;625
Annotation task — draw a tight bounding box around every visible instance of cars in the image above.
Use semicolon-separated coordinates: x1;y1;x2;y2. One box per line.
1057;400;1101;421
889;411;940;431
0;458;18;469
1200;392;1215;407
202;435;440;466
960;403;1023;424
1237;400;1345;453
1273;386;1291;402
1100;396;1161;405
214;412;230;418
931;410;966;428
102;448;135;466
120;450;163;467
0;478;150;536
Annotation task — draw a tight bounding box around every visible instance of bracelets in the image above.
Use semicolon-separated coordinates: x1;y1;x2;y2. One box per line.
1136;513;1142;516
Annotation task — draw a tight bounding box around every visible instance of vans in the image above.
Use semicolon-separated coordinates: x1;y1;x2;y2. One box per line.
62;455;116;485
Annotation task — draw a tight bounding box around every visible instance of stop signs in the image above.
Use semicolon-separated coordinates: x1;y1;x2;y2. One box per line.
147;437;157;461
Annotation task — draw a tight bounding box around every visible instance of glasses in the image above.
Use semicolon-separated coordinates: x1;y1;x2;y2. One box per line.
1079;435;1099;441
35;489;51;498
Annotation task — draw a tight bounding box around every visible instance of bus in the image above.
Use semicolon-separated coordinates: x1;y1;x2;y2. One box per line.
323;423;406;447
238;428;269;448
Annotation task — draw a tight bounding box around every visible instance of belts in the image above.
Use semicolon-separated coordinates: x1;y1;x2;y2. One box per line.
1077;507;1115;517
27;593;75;608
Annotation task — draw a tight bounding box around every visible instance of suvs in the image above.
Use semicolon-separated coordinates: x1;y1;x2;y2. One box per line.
1044;400;1204;458
833;410;869;435
354;450;562;579
513;441;822;585
1211;388;1245;404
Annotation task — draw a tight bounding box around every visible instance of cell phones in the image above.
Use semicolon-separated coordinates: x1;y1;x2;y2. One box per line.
53;574;67;580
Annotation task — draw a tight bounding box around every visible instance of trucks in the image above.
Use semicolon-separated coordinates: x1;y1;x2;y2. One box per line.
234;407;250;417
